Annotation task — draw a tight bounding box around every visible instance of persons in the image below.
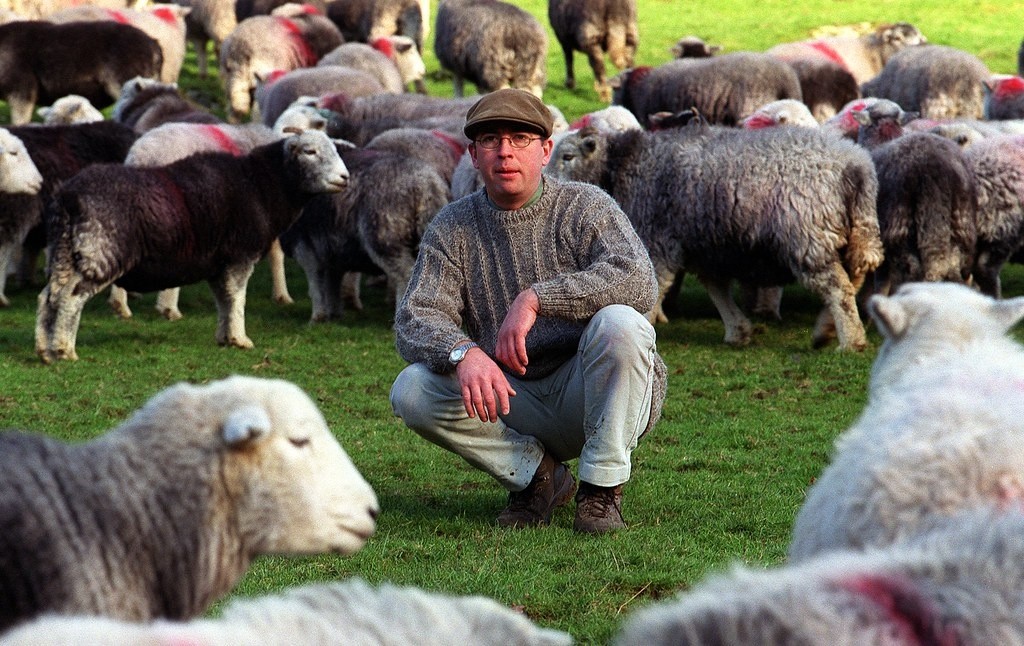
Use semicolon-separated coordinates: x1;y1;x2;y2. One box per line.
388;90;668;534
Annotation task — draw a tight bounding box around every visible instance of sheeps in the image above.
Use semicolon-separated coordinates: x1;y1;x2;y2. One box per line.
0;0;1024;646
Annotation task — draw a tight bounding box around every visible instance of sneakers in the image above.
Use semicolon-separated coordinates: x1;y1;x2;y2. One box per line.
573;474;624;533
494;451;578;531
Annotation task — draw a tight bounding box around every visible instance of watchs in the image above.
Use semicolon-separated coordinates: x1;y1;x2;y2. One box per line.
449;342;477;369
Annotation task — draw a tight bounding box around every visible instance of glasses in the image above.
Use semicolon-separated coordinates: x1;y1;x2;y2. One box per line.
470;131;545;149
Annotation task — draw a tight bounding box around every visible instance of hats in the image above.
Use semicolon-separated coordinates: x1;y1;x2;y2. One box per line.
464;88;554;142
